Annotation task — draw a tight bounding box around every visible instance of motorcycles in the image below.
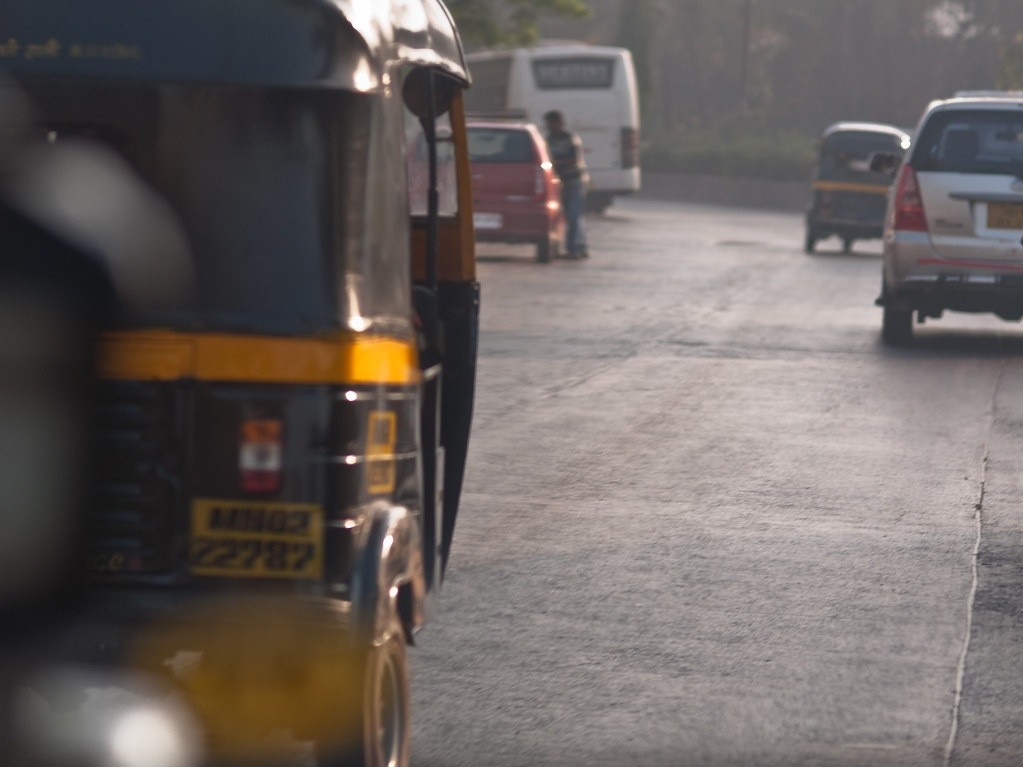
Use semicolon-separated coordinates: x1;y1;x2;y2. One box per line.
1;0;480;767
805;120;911;254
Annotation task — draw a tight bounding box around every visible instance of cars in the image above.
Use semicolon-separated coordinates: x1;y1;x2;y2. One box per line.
404;111;562;263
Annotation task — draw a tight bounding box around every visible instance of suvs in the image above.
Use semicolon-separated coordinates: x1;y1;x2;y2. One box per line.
869;91;1023;346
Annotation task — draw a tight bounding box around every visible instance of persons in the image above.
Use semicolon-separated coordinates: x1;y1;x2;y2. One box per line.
544;110;590;259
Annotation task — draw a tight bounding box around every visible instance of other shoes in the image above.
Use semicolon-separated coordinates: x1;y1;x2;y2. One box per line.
559;249;589;260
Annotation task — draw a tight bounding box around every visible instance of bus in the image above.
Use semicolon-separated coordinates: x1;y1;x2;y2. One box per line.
462;41;641;214
